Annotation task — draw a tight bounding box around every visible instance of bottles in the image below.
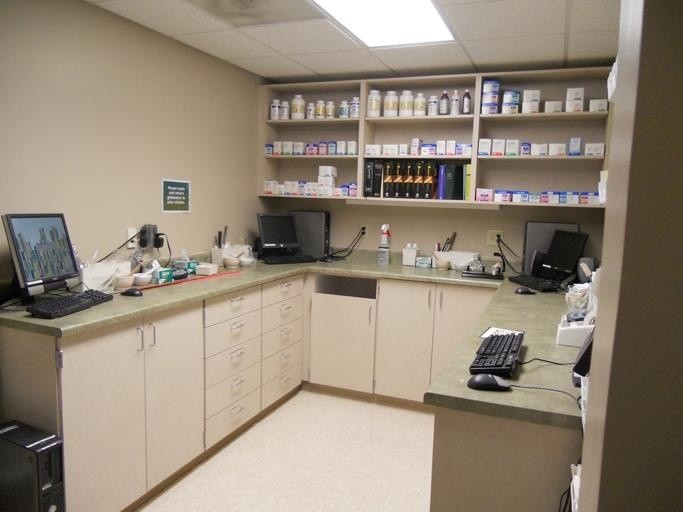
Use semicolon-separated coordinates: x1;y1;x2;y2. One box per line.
383;161;434;198
270;95;360;119
462;89;471;114
427;95;439;116
450;90;460;114
366;90;381;117
383;91;398;117
398;90;414;116
439;92;448;115
437;165;446;199
413;92;427;116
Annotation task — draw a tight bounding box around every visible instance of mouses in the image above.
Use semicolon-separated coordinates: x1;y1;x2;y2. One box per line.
324;256;333;263
121;288;142;297
467;373;512;393
515;287;531;294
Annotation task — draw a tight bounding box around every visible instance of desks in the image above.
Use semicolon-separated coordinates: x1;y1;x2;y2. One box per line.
423;280;584;511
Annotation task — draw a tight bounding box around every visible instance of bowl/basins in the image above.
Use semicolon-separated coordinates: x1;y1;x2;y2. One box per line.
223;258;238;268
111;275;134;291
131;273;151;286
239;258;252;267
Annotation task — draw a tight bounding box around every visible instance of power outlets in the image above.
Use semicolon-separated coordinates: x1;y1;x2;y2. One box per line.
486;230;503;245
359;222;368;238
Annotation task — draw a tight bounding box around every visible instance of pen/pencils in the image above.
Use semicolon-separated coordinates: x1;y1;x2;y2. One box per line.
72;245;99;269
218;231;221;249
434;240;438;251
442;238;449;252
223;226;228;245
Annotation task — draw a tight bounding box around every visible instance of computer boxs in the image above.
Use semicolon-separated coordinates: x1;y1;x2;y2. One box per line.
521;220;580;274
288;210;331;259
0;419;65;512
27;279;68;296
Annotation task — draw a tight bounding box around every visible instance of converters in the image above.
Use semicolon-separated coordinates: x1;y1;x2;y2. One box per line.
154;237;164;247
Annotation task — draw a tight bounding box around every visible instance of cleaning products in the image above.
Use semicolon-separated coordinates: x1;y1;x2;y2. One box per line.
378;223;392;248
378;249;390;267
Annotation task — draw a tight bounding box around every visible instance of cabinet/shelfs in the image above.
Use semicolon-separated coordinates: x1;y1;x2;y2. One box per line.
306;272;380;395
473;65;613;208
359;73;478;204
205;280;263;450
1;296;205;511
373;275;497;403
263;270;306;415
258;80;363;202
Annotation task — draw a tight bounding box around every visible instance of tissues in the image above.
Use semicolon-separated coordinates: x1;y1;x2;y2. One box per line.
174;248;199;275
144;258;173;284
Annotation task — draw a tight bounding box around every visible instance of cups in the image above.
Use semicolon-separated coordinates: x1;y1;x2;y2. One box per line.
565;285;588;320
435;256;449;271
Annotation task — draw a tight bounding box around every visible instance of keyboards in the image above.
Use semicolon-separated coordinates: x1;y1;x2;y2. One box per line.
508;274;558;292
263;255;319;264
469;332;524;375
25;289;113;319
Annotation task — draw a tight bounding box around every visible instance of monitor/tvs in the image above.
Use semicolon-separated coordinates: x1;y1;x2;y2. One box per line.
571;323;595;386
256;212;300;254
542;229;589;274
1;213;80;289
